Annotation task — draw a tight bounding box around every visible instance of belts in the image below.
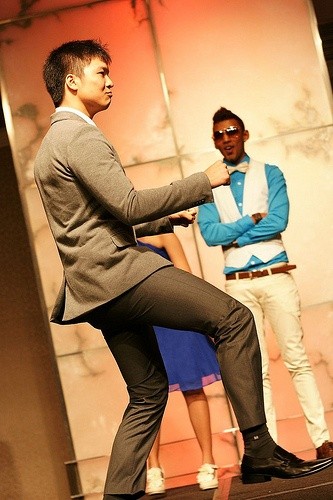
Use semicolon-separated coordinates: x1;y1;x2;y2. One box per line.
225;264;297;281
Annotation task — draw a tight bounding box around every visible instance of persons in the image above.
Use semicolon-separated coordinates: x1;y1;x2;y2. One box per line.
136;233;219;496
196;107;333;460
34;38;333;500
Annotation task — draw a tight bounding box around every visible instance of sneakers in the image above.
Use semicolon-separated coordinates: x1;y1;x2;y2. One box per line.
195;463;221;490
143;466;167;497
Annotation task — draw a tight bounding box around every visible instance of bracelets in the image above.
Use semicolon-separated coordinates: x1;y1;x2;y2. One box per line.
253;213;262;224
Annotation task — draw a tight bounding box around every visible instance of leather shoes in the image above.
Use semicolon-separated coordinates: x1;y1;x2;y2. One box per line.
239;445;333;485
101;495;146;500
315;440;333;460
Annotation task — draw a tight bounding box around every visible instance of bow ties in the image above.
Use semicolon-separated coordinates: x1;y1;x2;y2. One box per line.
226;161;249;175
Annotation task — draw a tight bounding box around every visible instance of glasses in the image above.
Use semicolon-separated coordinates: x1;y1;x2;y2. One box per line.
212;126;243;139
